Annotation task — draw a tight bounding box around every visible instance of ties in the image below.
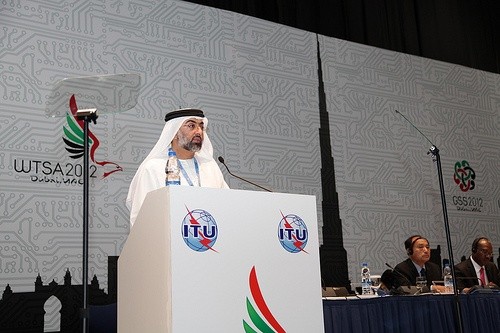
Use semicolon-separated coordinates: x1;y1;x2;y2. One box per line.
420;268;426;277
479;268;486;286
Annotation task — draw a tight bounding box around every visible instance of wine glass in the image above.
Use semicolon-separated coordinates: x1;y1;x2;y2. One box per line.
416;276;427;293
371;275;382;296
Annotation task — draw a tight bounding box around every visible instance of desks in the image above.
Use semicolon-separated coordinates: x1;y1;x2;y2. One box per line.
323;288;500;333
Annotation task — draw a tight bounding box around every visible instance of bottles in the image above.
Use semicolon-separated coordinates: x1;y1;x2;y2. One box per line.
165;151;180;186
443;263;454;293
361;263;371;294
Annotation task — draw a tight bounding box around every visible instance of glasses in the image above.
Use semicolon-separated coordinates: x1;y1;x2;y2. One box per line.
477;250;493;256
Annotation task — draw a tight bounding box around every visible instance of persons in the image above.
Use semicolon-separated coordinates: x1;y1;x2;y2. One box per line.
126;108;231;234
391;235;441;293
454;237;500;294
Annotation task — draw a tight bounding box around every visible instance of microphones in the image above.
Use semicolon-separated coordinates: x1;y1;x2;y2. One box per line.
454;266;490;293
386;263;420;295
218;157;272;191
